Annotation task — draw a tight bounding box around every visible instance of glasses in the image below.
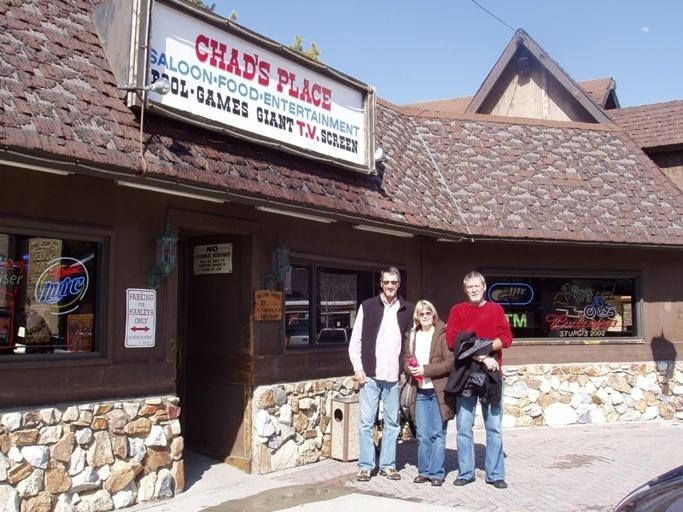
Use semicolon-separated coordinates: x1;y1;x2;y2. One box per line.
381;280;399;285
418;312;431;316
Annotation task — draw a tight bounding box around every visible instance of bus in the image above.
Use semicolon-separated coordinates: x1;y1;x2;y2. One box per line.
286;300;357;345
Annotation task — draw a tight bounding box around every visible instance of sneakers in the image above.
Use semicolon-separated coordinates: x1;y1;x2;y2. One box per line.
487;479;507;489
453;477;476;487
378;467;401;481
357;469;372;482
413;475;442;487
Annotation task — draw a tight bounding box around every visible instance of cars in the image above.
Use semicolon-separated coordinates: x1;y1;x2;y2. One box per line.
613;464;683;512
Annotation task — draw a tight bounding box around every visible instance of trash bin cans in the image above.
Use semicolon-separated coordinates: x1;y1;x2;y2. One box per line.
331;398;359;462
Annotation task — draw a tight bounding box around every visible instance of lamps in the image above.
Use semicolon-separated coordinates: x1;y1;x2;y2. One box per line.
263;238;286;298
147;218;177;289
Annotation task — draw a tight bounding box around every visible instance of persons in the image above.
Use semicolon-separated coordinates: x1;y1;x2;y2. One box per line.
444;270;508;489
346;265;448;481
400;298;456;487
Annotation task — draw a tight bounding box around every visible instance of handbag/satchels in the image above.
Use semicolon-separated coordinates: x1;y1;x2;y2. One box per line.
399;328;412;420
